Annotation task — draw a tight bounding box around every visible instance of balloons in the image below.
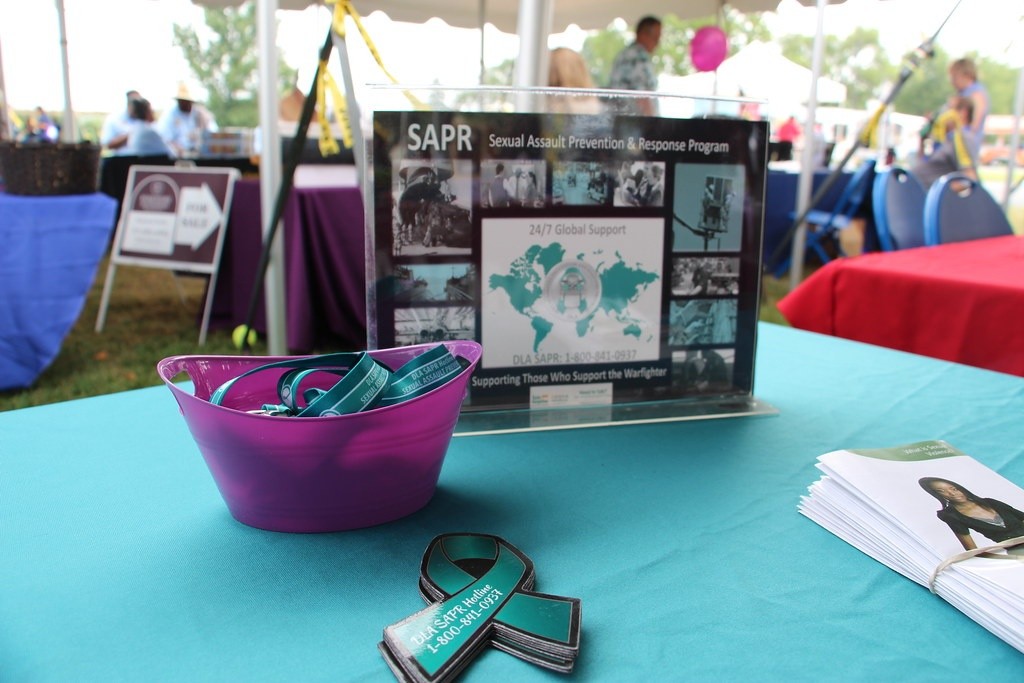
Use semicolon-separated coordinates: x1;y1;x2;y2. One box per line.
689;25;727;72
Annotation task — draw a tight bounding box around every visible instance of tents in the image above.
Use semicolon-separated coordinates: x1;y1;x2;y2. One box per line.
657;39;847;119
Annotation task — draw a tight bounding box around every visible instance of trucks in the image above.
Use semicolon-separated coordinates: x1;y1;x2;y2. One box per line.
816;105;928;159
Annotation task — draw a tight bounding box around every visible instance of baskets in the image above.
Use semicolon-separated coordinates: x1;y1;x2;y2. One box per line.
0;140;102;194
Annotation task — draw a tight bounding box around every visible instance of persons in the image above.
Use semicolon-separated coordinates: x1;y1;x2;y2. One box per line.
916;58;988;195
419;329;448;343
613;160;662;208
15;106;61;146
605;16;661;116
698;178;735;232
101;79;219;159
546;47;600;115
669;259;739;396
488;162;537;208
587;165;607;203
918;477;1024;560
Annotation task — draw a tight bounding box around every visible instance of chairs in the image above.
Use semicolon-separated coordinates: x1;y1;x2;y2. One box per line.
872;169;926;252
775;157;875;277
922;173;1013;246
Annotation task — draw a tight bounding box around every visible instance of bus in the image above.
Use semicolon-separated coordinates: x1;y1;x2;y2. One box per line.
981;114;1023;169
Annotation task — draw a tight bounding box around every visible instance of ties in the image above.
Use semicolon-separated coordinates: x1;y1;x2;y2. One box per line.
515;178;519;199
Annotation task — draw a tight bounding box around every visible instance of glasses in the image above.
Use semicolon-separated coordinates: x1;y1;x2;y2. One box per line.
398;276;408;281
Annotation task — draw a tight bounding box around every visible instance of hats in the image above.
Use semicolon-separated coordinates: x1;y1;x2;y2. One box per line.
172;83;197;103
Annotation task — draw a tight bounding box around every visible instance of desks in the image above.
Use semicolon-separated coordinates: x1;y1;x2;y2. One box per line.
0;144;1024;683
